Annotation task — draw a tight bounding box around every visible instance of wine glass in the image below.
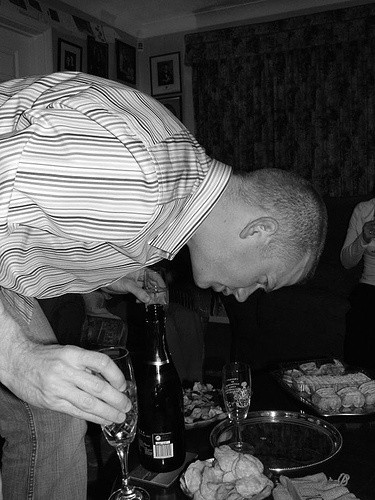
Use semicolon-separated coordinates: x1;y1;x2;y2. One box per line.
223;361;255;455
91;347;150;500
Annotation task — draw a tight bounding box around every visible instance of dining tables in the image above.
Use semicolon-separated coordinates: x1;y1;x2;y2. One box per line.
149;379;375;500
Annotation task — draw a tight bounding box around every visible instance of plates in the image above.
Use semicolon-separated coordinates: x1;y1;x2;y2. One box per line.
184;416;218;429
282;380;375;418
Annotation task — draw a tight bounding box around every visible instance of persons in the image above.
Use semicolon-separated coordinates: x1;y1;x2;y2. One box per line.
0;71;329;500
340;195;375;429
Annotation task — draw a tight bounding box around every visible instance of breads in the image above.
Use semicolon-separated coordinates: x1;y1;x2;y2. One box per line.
181;445;267;500
281;358;375;411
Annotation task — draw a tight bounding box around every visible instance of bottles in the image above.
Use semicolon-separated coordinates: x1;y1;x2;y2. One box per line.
137;304;186;473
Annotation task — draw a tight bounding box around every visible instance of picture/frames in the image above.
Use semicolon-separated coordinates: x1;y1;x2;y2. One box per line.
87;34;109;80
149;51;182;97
155;95;182;123
115;39;137;85
58;37;83;72
